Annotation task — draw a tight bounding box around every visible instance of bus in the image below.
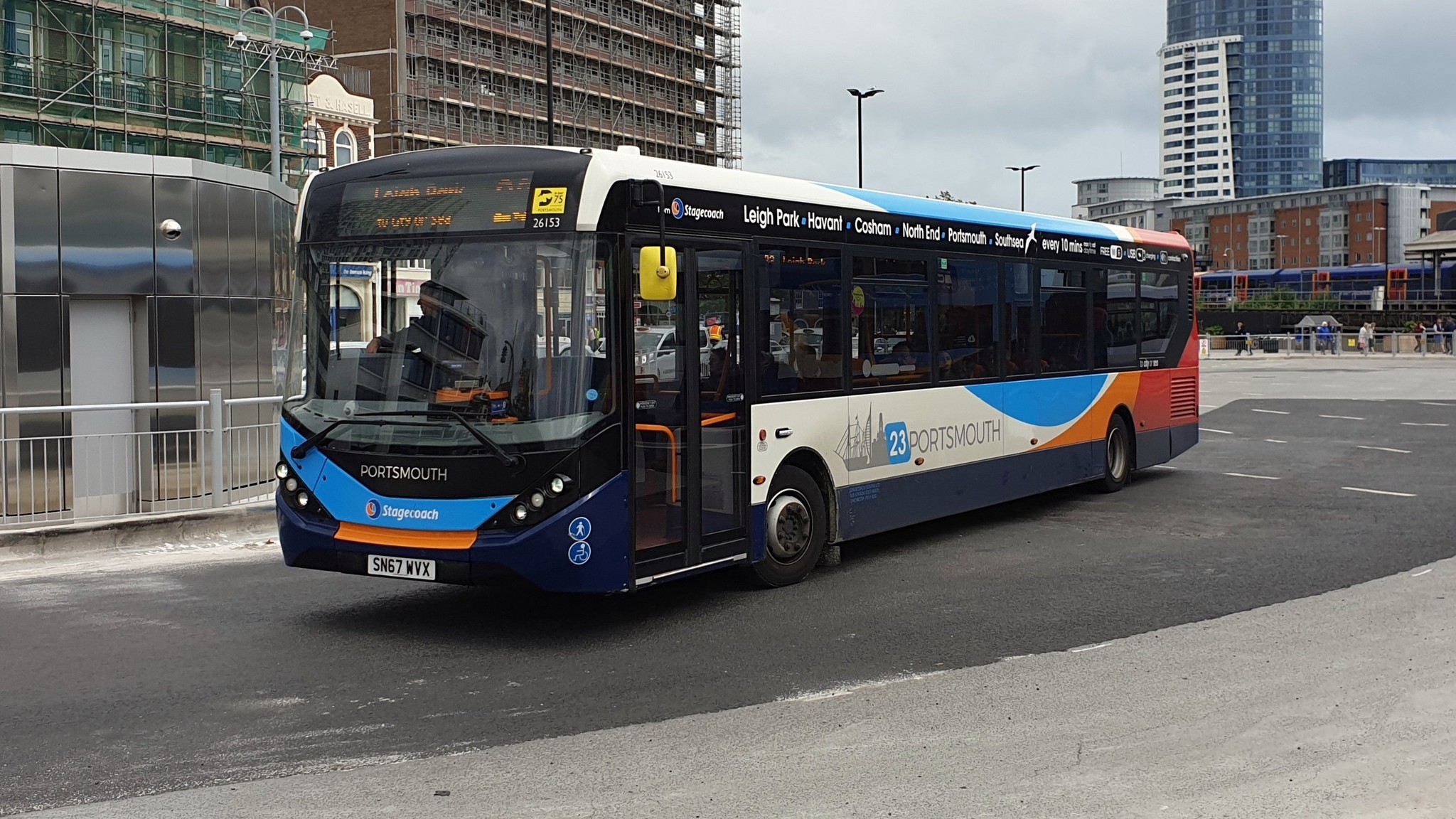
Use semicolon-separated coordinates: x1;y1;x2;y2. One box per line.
274;142;1201;612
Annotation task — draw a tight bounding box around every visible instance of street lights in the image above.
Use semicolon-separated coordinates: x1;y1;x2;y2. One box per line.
1004;165;1040;212
1274;234;1286;268
1374;227;1386;263
846;89;884;189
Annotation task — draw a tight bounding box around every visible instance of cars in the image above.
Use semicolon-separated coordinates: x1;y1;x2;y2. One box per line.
476;328;912;383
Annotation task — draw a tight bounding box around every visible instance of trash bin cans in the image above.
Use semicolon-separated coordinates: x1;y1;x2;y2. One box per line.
770;319;782;351
770;298;781;315
1263;340;1278;352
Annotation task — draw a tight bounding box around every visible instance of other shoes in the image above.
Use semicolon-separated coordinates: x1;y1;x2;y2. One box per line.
1443;352;1447;354
1236;353;1241;355
1332;353;1336;354
1373;352;1377;354
1431;352;1435;354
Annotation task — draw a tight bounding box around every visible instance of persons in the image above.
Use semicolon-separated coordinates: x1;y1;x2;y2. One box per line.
708;317;726;347
1325;321;1335;354
1093;292;1114;368
1357;321;1376;351
367;280;476;391
935;288;954;331
588;326;600;352
938;344;1018;379
1415;316;1456;354
892;341;916;367
701;347;734;391
1041;351;1079;373
760;344;833;395
1235;322;1247;354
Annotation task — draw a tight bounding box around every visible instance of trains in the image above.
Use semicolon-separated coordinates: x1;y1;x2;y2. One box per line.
1194;262;1456;325
